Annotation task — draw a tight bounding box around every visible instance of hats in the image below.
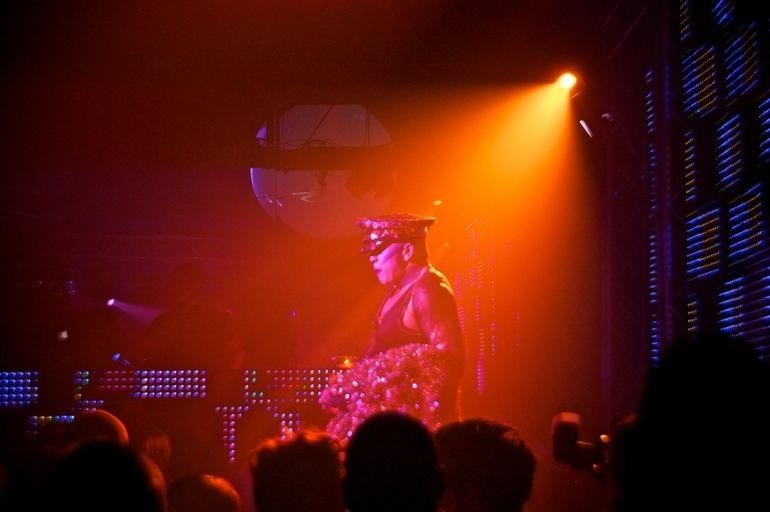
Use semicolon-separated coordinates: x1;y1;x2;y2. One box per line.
351;212;436;256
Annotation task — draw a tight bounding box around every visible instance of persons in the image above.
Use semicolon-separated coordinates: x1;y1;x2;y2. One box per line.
357;213;468;422
0;331;769;511
